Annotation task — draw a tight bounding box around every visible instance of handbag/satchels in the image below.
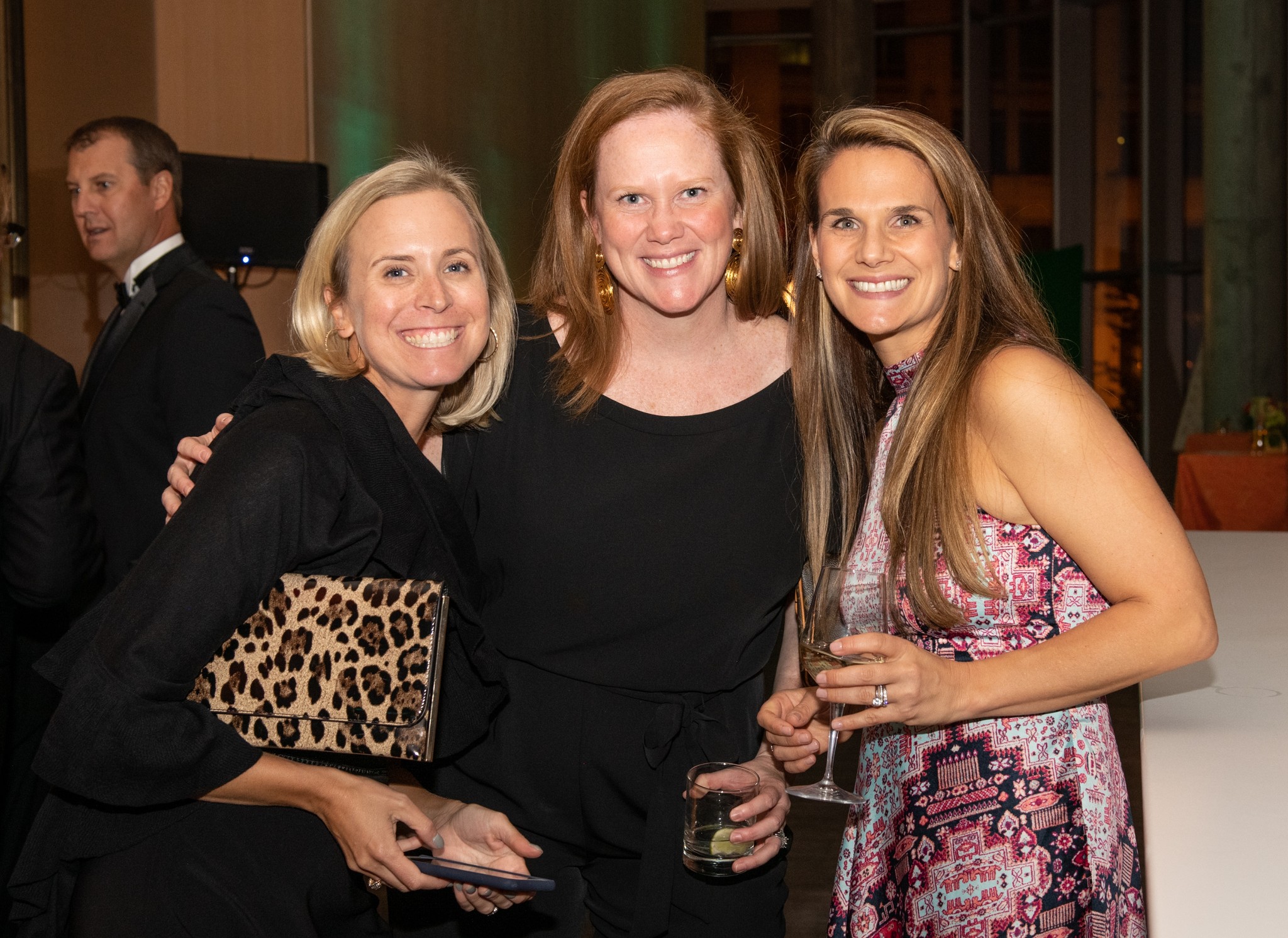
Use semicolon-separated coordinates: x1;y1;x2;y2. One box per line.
186;573;450;763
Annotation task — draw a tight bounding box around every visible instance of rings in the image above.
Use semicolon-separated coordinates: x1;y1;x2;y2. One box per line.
770;742;774;752
764;828;789;849
873;684;889;708
369;877;382;890
486;906;498;916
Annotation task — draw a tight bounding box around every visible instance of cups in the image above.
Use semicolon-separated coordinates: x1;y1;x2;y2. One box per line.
683;760;759;878
1250;419;1270;455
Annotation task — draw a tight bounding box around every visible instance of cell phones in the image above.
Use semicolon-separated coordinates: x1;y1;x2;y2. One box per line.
402;856;556;891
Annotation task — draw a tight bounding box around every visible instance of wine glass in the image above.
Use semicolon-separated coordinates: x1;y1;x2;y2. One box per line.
785;568;889;805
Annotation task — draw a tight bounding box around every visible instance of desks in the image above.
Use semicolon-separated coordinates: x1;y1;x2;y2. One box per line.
1174;431;1288;530
1140;529;1287;938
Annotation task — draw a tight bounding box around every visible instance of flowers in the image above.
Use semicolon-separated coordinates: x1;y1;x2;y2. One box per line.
1239;395;1286;428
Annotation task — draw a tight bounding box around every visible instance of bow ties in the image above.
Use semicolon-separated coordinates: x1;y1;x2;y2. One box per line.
113;282;132;309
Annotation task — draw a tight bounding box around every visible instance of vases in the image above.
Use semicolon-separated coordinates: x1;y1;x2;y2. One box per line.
1249;423;1269;453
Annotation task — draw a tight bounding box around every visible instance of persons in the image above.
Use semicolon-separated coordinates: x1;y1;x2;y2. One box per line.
0;321;78;722
60;112;268;623
40;148;547;938
755;107;1223;938
158;68;855;938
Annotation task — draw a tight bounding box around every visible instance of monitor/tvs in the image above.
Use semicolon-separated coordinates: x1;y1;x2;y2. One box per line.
178;150;329;268
